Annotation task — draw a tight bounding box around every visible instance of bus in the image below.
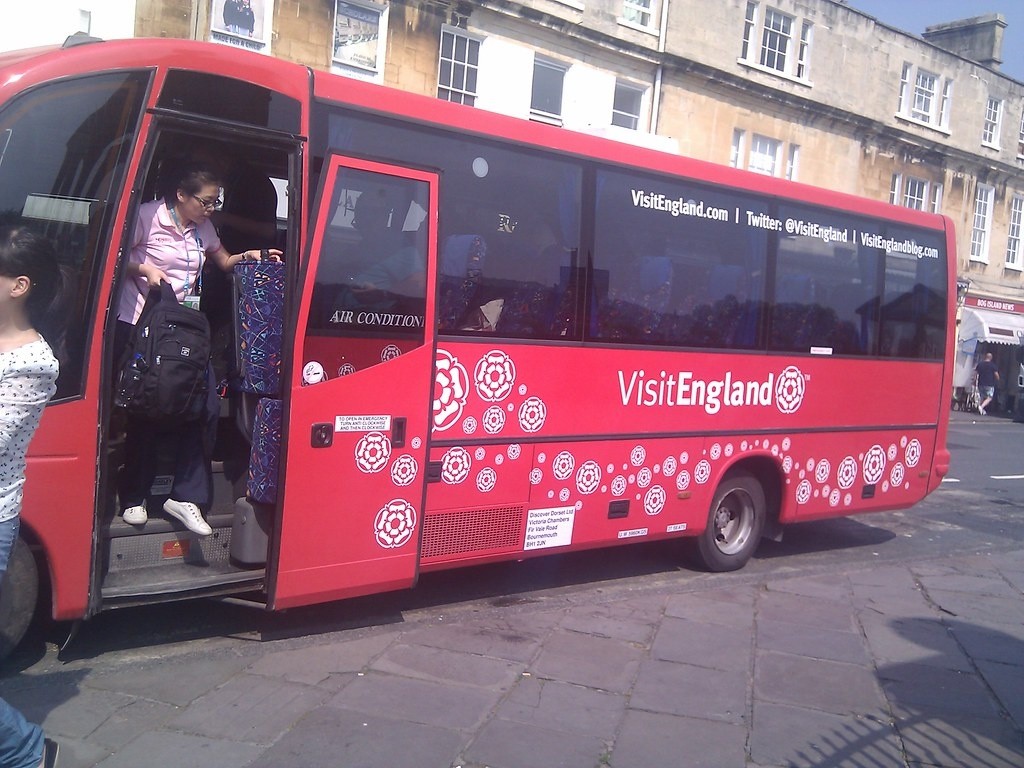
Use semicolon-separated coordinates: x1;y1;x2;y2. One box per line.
0;36;957;664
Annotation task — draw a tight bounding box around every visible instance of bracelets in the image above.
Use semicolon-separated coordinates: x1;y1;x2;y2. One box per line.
242;250;248;260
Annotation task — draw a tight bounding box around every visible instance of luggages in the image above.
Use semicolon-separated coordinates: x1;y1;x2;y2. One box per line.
234;250;286;395
246;398;283;505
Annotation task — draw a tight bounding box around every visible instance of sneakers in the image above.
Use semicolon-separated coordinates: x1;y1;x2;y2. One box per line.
123;506;148;525
162;498;212;536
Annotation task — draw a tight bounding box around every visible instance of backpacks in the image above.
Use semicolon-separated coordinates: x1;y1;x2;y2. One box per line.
125;279;213;432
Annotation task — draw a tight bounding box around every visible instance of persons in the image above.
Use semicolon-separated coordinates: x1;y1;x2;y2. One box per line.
0;223;62;768
972;352;1000;414
223;0;255;40
189;136;280;396
325;187;426;329
113;162;285;538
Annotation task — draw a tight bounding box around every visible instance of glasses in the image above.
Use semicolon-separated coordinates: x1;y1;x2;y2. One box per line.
351;218;362;229
193;195;223;208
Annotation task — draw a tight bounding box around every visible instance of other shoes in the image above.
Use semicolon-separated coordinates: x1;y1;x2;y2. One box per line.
979;406;986;416
44;738;60;768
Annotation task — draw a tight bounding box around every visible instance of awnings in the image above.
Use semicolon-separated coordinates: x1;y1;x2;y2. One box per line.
957;306;1024;347
